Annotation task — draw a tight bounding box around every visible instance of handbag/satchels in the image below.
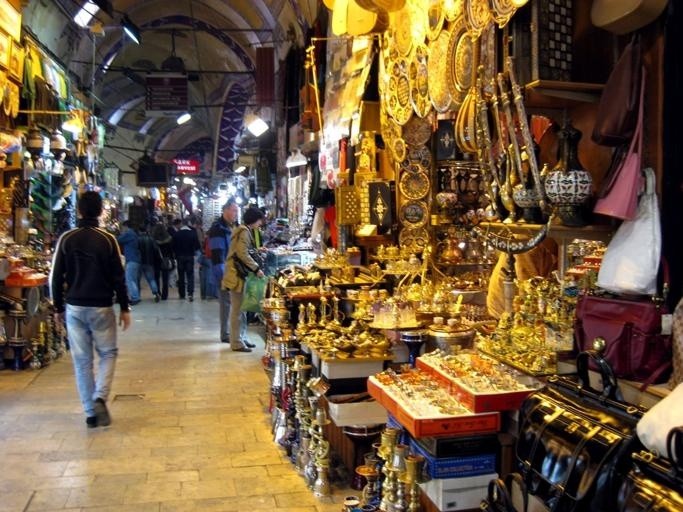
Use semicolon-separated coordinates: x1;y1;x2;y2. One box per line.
240;272;268;313
571;33;677;388
231;228;264;278
479;350;682;512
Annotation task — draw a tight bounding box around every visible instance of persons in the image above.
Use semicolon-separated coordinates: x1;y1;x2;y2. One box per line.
117;215;206;306
49;191;132;428
219;209;267;351
208;203;238;342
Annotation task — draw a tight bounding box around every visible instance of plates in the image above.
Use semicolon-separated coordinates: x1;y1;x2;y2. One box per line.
399;200;429;229
391;138;406;163
400;143;433;174
377;0;531;151
399;226;429;254
398;170;430;200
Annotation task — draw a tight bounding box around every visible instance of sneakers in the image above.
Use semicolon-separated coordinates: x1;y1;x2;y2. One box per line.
231;342;256;352
85;398;110;427
